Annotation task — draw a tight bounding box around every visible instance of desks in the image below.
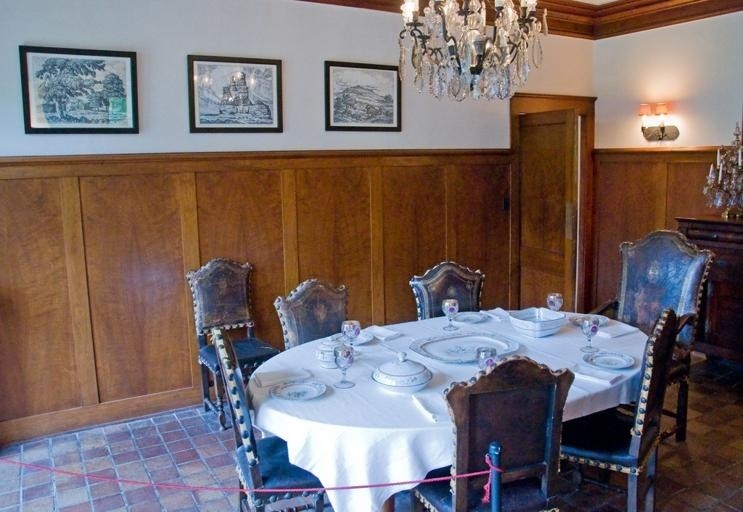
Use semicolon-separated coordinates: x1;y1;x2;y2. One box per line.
673;213;743;384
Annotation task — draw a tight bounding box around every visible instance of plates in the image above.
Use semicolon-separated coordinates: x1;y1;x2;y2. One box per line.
370;370;434;394
569;314;607;327
454;310;487;325
407;331;520;364
582;352;634;371
268;379;325;402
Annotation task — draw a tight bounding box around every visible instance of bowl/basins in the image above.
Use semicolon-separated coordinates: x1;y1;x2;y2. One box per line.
509;307;566;338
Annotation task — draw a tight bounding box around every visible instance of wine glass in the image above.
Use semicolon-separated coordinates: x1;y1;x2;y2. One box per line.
579;318;599;354
335;346;355;390
341;321;358;356
442;299;460;332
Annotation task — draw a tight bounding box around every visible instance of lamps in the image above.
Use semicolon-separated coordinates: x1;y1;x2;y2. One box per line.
702;122;743;219
638;102;680;141
400;1;549;104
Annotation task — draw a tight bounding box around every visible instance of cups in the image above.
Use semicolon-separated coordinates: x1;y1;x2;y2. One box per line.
548;293;563;314
475;346;495;369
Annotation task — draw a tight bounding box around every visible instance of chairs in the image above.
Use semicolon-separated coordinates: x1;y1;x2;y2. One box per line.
407;258;486;321
185;257;280;431
212;326;332;512
558;306;676;510
412;354;576;511
585;229;715;443
274;277;349;350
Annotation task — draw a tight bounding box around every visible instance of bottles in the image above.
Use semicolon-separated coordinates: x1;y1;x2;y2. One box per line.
315;335;341;369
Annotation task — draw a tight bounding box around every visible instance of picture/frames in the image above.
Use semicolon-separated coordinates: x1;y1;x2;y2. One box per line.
186;53;283;133
323;60;402;132
18;44;139;135
